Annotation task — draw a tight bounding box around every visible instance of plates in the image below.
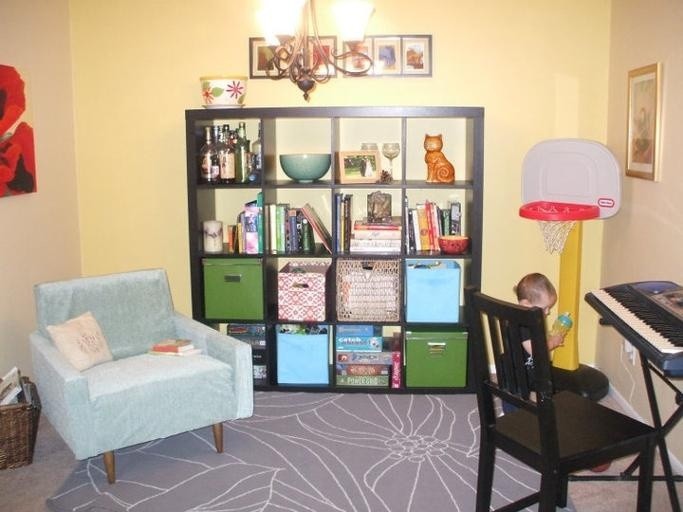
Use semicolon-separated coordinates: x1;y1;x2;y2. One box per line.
201;104;246;110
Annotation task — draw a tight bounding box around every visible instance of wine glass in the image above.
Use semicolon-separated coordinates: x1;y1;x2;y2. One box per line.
382;142;401;185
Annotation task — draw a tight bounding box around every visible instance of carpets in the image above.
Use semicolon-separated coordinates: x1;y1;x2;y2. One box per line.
41;389;578;511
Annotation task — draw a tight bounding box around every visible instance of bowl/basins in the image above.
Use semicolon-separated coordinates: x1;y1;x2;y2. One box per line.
437;235;469;255
199;75;248;104
280;153;331;184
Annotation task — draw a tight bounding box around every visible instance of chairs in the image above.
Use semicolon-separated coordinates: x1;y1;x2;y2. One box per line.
30;268;243;479
467;289;654;508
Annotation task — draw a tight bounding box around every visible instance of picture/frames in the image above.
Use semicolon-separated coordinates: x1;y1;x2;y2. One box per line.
624;61;664;184
278;37;307;79
341;34;374;77
339;149;383;184
307;35;337;78
398;34;431;78
372;34;401;78
248;36;279;80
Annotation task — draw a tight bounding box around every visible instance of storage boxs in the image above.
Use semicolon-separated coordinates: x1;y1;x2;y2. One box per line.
276;326;329;386
202;257;264;320
404;260;460;325
403;331;467;389
277;259;326;321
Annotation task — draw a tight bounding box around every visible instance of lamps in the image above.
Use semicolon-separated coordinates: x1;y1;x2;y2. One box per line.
252;2;374;105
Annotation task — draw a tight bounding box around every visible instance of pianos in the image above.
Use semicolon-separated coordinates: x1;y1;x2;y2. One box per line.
584;280;682;379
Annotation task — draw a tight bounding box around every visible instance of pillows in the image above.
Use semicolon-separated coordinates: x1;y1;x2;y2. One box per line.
46;311;114;372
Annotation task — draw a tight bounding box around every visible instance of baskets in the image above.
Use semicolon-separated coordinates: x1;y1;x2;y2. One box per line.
335;257;400;322
0;376;42;471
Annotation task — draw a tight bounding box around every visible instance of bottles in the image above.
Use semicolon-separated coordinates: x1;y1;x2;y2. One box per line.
550;313;572;348
200;123;263;182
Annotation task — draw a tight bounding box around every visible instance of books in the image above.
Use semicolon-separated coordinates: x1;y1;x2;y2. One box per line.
148;348;200;357
225;192;467;259
0;365;33;406
153;338;193;352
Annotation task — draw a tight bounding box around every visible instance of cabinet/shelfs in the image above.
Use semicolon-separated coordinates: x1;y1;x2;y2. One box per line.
184;109;484;391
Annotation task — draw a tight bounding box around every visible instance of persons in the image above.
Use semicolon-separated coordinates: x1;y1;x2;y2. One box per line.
508;272;565;405
633;107;653;163
